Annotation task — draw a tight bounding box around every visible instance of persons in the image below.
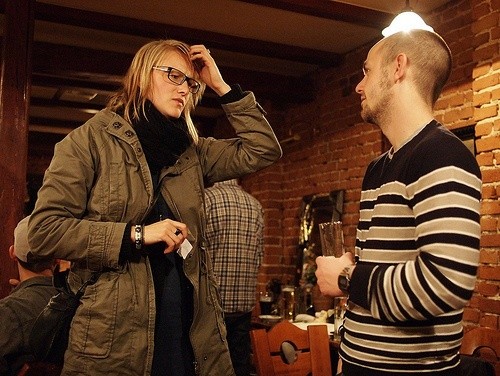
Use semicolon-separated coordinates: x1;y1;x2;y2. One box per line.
28;38;284;376
0;215;75;376
203;175;265;376
315;29;483;376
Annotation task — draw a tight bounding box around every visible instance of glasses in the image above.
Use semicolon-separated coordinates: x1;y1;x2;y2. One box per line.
151;66;201;94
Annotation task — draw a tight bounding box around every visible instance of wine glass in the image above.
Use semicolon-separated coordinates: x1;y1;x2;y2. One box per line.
318;221;349;258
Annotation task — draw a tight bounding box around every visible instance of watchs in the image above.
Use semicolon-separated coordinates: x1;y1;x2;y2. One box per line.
337;264;354;295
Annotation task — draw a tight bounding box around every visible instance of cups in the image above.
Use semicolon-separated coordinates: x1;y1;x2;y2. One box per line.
281;284;295;322
259;291;272;315
332;296;347;342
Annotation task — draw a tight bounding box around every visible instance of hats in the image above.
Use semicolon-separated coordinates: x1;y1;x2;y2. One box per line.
13;214;46;263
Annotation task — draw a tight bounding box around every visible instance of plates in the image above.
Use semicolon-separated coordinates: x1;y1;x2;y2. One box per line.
259;315;282;322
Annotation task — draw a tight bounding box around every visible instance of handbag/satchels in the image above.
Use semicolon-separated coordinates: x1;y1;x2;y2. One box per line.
28;286;79;364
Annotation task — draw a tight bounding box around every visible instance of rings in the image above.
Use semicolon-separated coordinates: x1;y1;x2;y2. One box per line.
175;229;181;236
207;48;210;53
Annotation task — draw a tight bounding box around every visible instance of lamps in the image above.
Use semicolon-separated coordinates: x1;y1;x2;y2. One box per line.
384;1;435;37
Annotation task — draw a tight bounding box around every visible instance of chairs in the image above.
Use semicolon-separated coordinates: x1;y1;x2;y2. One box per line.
249;319;332;376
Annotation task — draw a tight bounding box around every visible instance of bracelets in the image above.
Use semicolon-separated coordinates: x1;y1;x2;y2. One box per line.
135;224;143;251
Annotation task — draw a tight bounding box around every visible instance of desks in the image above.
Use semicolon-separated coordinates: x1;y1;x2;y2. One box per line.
253;311;342;348
